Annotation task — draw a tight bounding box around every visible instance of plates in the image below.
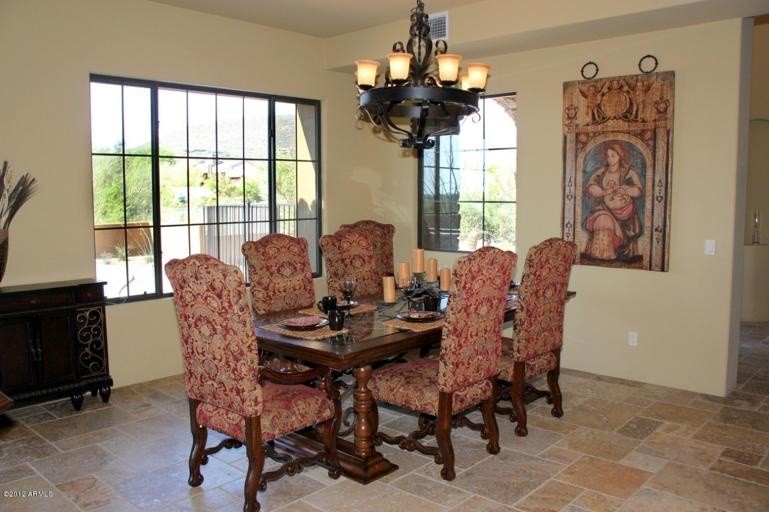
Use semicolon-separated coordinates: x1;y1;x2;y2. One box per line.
396;311;444;323
275;317;329;329
337;299;359;309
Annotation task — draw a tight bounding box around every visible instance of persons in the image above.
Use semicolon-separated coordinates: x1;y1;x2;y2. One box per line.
582;141;643;263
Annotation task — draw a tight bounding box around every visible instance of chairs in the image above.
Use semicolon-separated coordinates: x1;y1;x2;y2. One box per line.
161;254;342;512
242;231;332;387
360;245;519;482
493;237;578;437
318;219;398;366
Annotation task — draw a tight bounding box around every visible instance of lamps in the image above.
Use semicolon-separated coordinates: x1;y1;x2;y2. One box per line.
353;1;492;155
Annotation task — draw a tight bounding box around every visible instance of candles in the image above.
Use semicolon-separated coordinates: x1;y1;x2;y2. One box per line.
424;258;437;282
412;249;426;274
440;268;450;290
397;261;409;286
380;276;396;303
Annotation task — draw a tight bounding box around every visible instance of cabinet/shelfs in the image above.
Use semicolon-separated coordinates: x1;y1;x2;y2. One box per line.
1;277;115;414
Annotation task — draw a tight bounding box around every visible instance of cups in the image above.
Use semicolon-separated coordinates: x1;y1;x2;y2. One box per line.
328;311;345;331
317;296;337;315
424;299;438;311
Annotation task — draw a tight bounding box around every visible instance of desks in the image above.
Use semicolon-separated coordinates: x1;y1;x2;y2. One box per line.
250;281;580;485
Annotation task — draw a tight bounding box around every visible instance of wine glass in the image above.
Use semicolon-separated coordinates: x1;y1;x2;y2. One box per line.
340;275;356;318
